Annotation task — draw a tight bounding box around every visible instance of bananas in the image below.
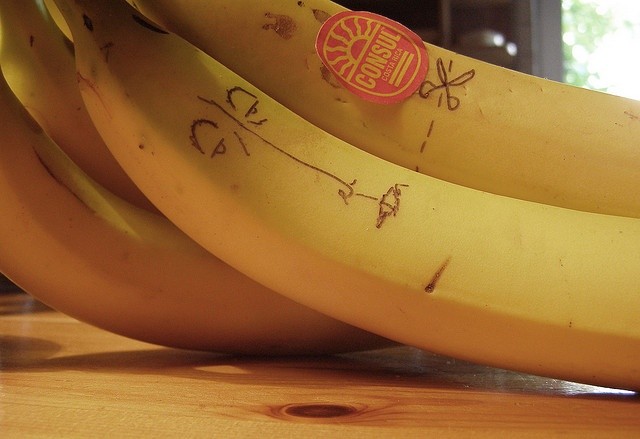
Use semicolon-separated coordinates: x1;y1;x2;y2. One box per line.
1;0;639;373
